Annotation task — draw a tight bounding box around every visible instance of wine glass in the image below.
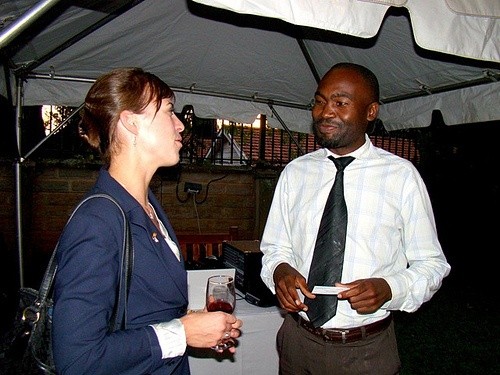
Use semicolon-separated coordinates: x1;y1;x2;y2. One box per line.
206;275;236;350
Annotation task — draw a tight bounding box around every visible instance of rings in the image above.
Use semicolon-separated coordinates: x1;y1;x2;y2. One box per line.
225;332;230;338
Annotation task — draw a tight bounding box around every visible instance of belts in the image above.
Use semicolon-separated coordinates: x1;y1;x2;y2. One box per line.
290;313;393;343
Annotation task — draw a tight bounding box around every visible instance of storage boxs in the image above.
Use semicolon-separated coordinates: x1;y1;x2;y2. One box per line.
222;240;266;292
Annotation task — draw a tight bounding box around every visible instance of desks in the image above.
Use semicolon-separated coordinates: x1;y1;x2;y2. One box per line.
187;269;284;375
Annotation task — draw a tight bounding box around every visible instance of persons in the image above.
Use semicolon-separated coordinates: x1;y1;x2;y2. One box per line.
260;62;452;374
52;66;243;375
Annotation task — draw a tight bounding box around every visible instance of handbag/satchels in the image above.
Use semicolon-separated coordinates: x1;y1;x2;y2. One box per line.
0;193;133;375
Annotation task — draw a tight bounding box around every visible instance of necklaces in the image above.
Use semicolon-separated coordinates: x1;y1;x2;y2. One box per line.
143;204;155;221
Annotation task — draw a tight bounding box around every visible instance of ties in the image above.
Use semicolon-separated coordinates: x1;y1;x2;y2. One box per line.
303;155;356;328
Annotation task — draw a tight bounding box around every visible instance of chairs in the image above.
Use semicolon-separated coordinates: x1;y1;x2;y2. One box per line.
175;227;238;261
